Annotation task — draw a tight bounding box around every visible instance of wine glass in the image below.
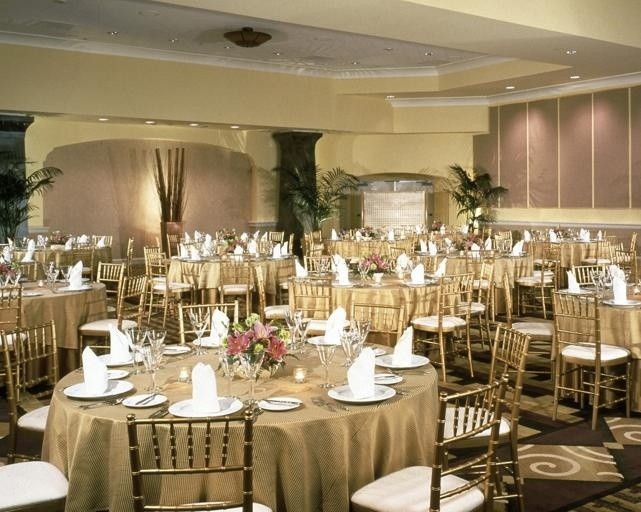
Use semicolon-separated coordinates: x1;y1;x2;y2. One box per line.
526;226;609;243
328;224;468;243
416;237;528;259
123;303;371;407
5;232;107;251
0;261;92;297
174;231;291;265
291;256;449;288
588;264;641;308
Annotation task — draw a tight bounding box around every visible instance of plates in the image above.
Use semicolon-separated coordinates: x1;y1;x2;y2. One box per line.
168;397;244;417
374;353;429;370
374;373;403;385
373;347;386;357
97;352;145;367
106;370;129;379
328;384;396;403
63;380;133;398
257;396;302;411
559;288;591;297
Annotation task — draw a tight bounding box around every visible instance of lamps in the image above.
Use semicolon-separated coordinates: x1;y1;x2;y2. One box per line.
223;27;272;48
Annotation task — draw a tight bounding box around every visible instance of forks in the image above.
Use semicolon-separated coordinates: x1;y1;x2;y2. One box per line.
79;396;126;410
